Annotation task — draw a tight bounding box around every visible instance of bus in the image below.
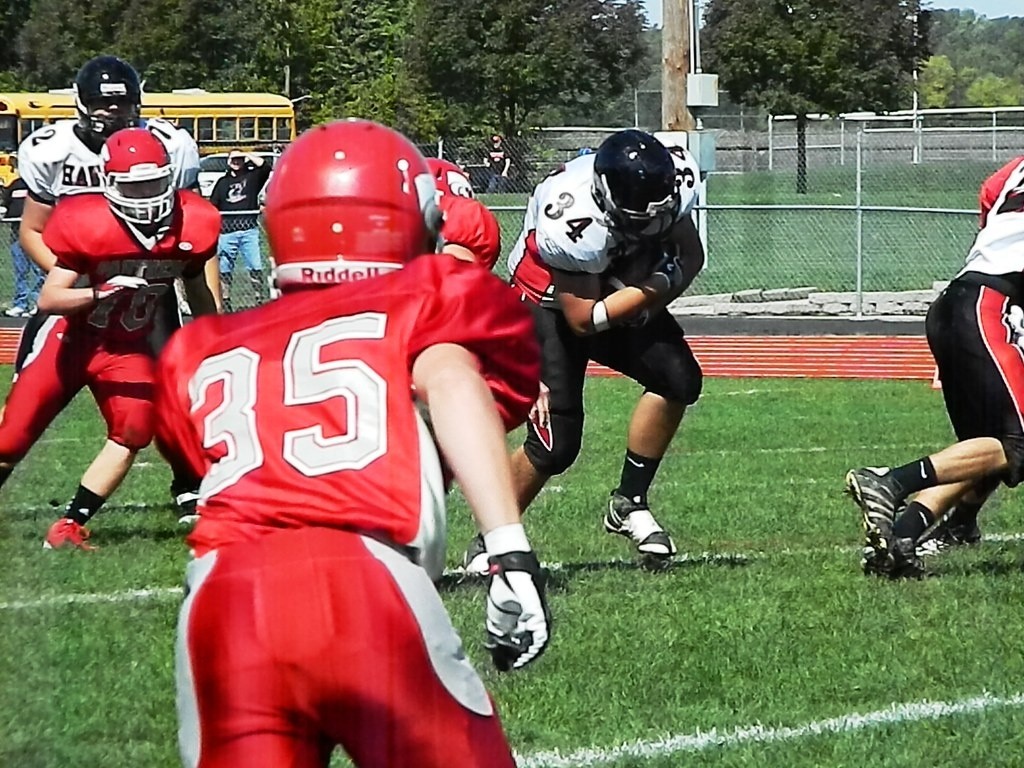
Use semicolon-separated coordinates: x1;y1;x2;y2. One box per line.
0;87;297;188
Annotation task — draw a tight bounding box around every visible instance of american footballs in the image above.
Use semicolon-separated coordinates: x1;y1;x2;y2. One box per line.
600;239;675;300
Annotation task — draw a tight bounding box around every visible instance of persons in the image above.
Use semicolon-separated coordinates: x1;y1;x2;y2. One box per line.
463;129;703;579
483;133;511;193
209;149;272;312
845;155;1024;579
0;56;224;552
150;114;551;768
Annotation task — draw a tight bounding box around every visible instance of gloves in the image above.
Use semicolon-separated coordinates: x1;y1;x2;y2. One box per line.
94;276;149;302
486;550;550;671
652;243;685;291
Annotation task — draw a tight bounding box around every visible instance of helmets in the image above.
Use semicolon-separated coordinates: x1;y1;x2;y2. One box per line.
426;158;475;208
75;56;141;140
594;130;681;238
265;120;435;283
99;128;174;224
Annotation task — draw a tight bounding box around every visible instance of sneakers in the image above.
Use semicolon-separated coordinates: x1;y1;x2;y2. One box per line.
603;495;678;555
43;518;97;550
846;465;907;556
461;535;489;572
915;524;981;556
176;492;201;524
860;536;926;577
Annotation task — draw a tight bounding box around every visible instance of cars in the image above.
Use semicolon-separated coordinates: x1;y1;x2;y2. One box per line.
193;150;284;199
